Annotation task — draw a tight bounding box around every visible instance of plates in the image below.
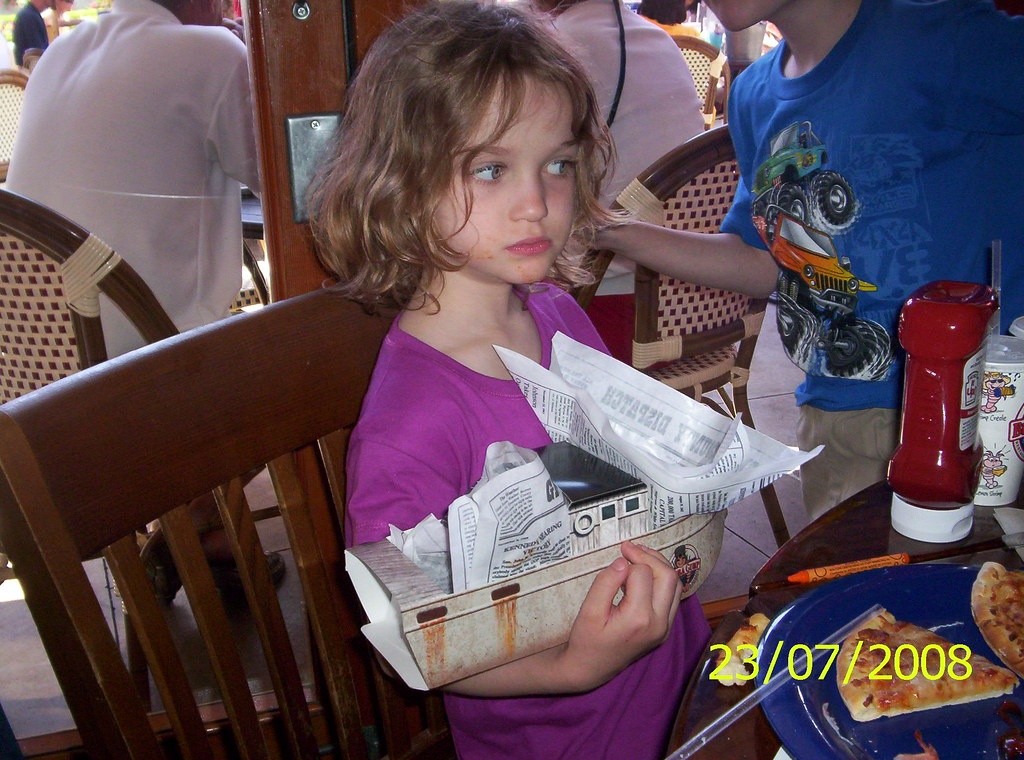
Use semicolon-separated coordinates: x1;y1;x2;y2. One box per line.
754;563;1024;760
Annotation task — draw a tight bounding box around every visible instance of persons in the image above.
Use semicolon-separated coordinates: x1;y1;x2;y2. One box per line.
0;0;1024;613
309;0;713;760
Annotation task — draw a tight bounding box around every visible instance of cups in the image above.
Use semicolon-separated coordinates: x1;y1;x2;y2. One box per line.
726;20;768;66
972;335;1024;507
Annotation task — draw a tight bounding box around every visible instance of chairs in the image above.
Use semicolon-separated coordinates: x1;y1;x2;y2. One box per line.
0;285;454;760
0;190;180;712
569;125;791;550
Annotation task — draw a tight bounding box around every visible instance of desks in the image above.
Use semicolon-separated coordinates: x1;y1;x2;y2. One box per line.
664;477;1024;760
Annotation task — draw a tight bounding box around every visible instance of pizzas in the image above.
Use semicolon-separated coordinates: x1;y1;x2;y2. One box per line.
715;612;772;686
836;607;1019;722
970;561;1024;682
